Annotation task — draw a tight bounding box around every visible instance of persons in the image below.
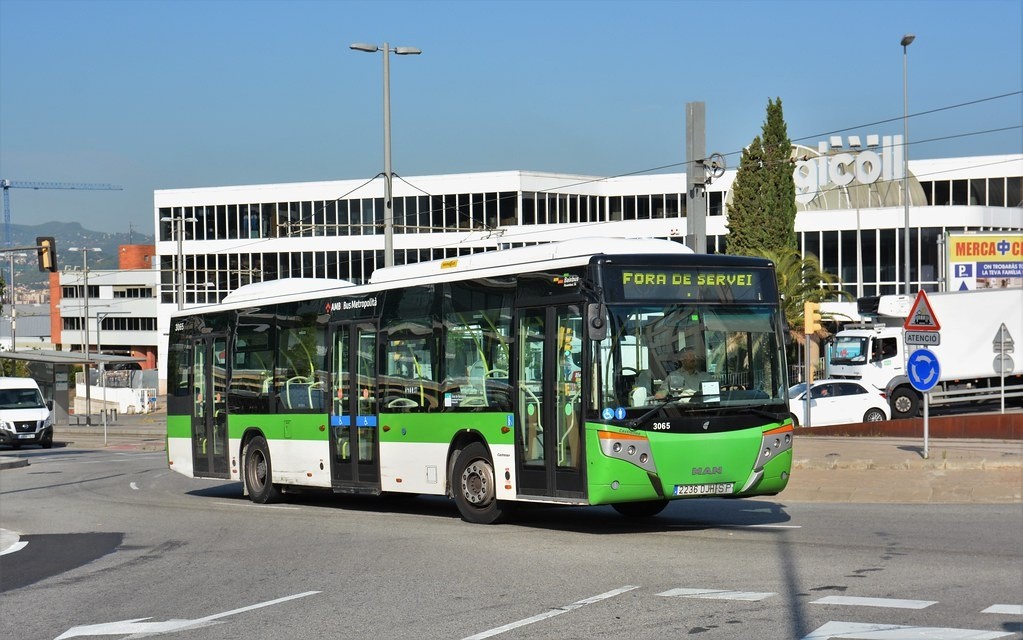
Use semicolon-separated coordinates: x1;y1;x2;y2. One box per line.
819;386;830;397
660;347;745;394
871;342;892;361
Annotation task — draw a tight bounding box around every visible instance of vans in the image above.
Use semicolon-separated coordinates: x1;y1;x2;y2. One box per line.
0;375;55;449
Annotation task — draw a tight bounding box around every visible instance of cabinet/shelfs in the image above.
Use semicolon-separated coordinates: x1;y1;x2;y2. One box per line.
263;374;510;412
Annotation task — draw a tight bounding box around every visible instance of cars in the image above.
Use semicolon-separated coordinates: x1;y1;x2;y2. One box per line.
780;377;892;429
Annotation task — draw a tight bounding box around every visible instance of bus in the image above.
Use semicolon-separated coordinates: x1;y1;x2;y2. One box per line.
162;234;795;527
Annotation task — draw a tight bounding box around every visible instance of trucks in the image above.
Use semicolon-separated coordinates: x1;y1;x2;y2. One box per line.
822;286;1023;420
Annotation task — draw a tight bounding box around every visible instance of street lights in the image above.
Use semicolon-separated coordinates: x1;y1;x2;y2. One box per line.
9;251;28;376
344;39;423;270
66;247;104;426
900;32;916;293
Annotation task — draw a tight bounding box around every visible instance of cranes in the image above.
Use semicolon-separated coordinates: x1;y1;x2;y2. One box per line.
0;178;124;247
